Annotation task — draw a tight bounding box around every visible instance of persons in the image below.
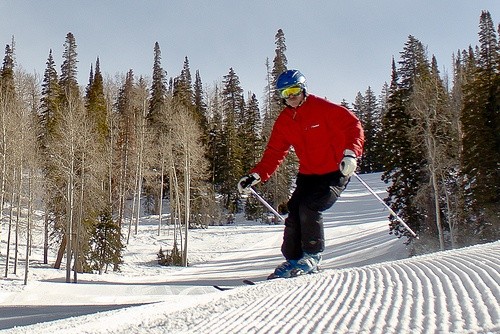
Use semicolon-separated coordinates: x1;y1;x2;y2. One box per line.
238;69;363;276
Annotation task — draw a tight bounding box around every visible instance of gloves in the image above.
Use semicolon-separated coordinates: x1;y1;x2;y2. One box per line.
339;149;357;177
238;172;261;193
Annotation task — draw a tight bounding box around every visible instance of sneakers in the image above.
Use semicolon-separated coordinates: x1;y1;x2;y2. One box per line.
274;260;296;278
290;253;322;275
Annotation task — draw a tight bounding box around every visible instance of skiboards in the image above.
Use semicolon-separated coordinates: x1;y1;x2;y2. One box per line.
213;280;271;291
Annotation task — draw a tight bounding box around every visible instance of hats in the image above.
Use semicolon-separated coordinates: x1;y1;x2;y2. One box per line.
277;70;307;104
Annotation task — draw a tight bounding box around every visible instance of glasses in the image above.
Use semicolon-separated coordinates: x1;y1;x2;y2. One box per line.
279;87;303;98
